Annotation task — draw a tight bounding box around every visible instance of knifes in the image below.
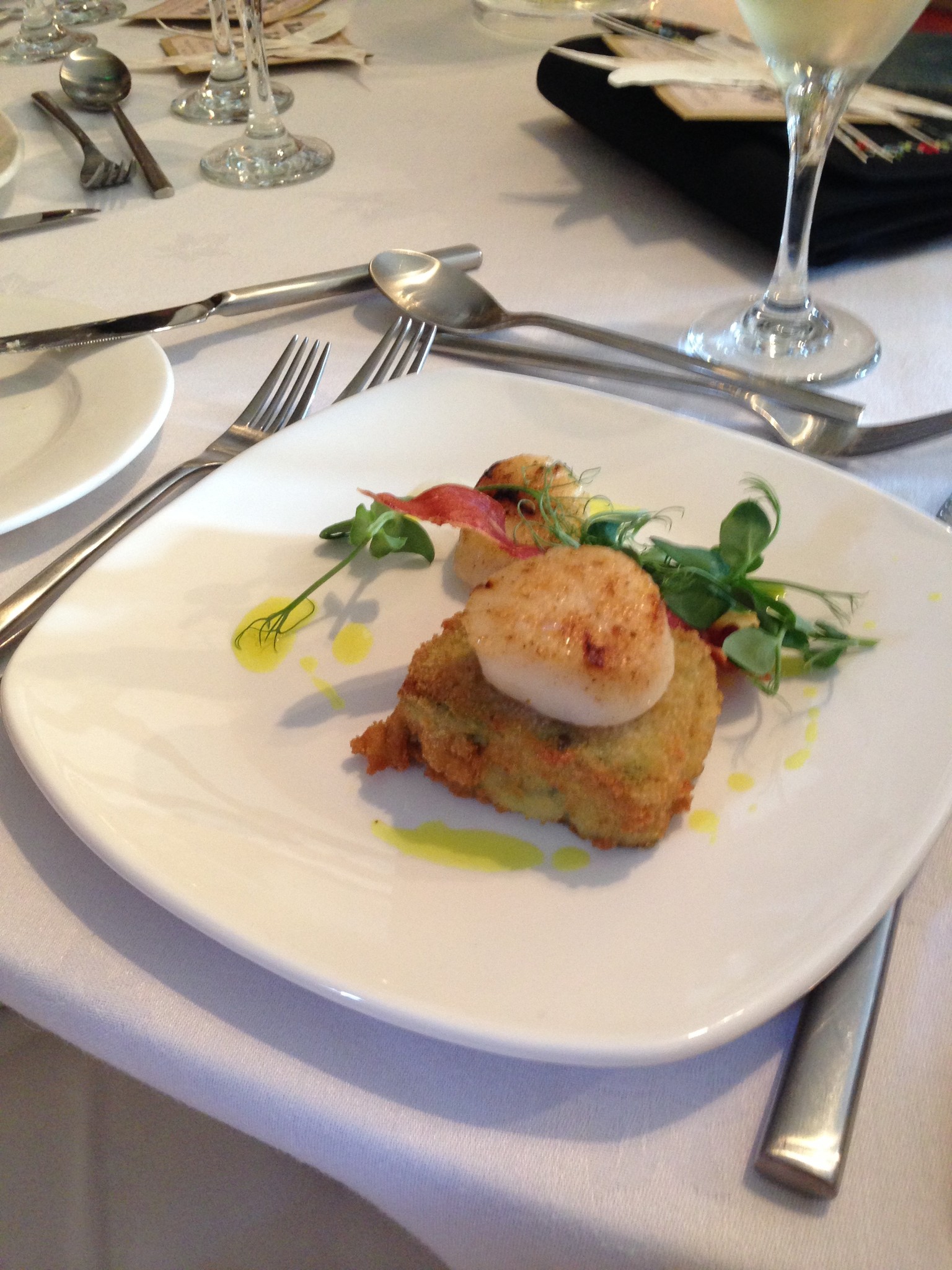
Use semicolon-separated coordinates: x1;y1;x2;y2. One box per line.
0;208;103;237
0;245;481;351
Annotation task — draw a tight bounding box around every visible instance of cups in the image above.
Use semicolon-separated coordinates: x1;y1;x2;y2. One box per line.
471;1;659;49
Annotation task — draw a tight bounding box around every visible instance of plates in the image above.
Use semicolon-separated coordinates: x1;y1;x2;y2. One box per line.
0;357;951;1074
2;293;176;537
0;112;24;188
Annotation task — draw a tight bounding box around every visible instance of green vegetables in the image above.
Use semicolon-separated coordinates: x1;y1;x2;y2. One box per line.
231;461;881;698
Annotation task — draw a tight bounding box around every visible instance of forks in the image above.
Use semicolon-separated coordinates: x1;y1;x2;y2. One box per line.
1;332;330;661
408;319;952;457
33;91;138;190
333;314;436;405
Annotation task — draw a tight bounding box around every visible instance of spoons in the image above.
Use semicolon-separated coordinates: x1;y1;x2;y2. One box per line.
58;47;176;200
364;247;871;426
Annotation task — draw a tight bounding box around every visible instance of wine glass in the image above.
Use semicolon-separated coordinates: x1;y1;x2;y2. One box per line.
679;0;941;386
172;0;336;189
0;0;127;63
